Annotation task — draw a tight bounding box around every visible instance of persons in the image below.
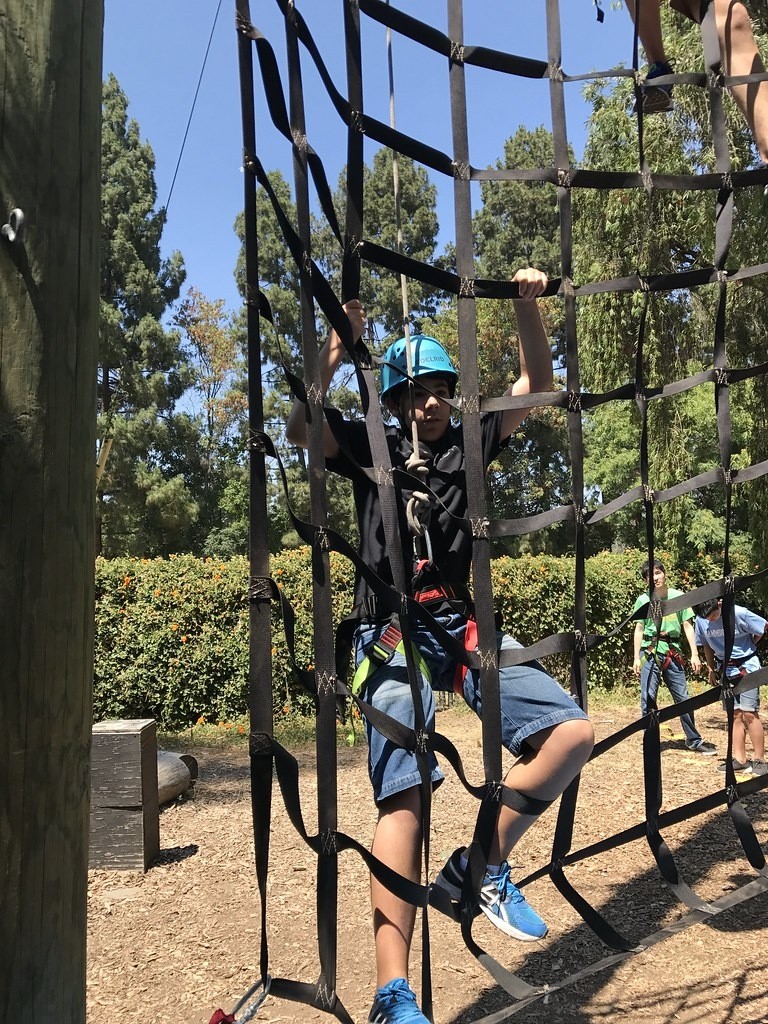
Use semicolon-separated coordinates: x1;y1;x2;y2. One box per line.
624;0;768;164
285;266;595;1024
632;560;768;775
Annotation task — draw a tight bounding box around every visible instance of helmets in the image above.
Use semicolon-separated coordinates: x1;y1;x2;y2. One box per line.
380;335;458;407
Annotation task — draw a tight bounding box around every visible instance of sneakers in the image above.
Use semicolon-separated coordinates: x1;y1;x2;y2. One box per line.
686;744;717;755
368;977;431;1024
752;759;768;778
435;846;548;942
717;758;753;774
